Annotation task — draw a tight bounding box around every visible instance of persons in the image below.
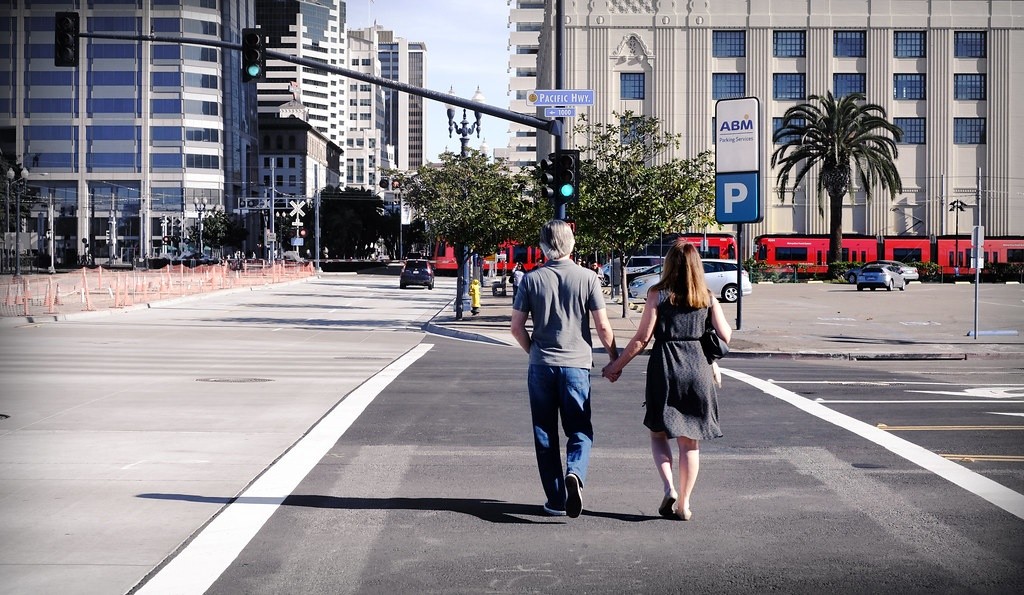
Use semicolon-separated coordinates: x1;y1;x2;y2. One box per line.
531;258;543;271
602;242;733;519
577;259;604;278
511;220;623;516
512;263;526;303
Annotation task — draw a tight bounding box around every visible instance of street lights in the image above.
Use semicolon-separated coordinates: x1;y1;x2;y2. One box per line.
194;196;207;256
4;172;48;273
949;198;967;267
445;82;486;312
276;210;286;261
7;165;30;275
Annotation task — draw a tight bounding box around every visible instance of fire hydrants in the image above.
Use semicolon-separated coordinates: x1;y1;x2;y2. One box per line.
469;278;482;316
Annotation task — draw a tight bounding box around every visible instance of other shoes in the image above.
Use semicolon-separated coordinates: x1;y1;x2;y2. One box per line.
676;507;692;520
659;489;678;516
544;503;566;516
565;473;583;518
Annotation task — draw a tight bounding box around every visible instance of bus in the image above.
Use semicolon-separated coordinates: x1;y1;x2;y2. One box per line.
432;235;548;272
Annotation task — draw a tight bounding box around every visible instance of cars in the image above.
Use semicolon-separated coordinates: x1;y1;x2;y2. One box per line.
845;261;919;292
405;252;422;260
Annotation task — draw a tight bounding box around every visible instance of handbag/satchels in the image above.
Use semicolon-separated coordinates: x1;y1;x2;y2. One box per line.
509;271;515;283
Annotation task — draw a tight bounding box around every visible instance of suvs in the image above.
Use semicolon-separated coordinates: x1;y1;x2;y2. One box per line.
400;260;437;288
603;255;752;301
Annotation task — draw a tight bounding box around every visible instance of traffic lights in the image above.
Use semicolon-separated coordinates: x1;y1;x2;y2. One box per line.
171;236;180;244
301;230;306;236
240;27;266;82
539;149;581;205
248;201;253;207
239;200;246;208
379;179;388;188
54;10;80;66
162;236;170;243
391;179;401;188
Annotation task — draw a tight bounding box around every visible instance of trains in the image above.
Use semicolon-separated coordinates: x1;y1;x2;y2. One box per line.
663;233;1024;280
